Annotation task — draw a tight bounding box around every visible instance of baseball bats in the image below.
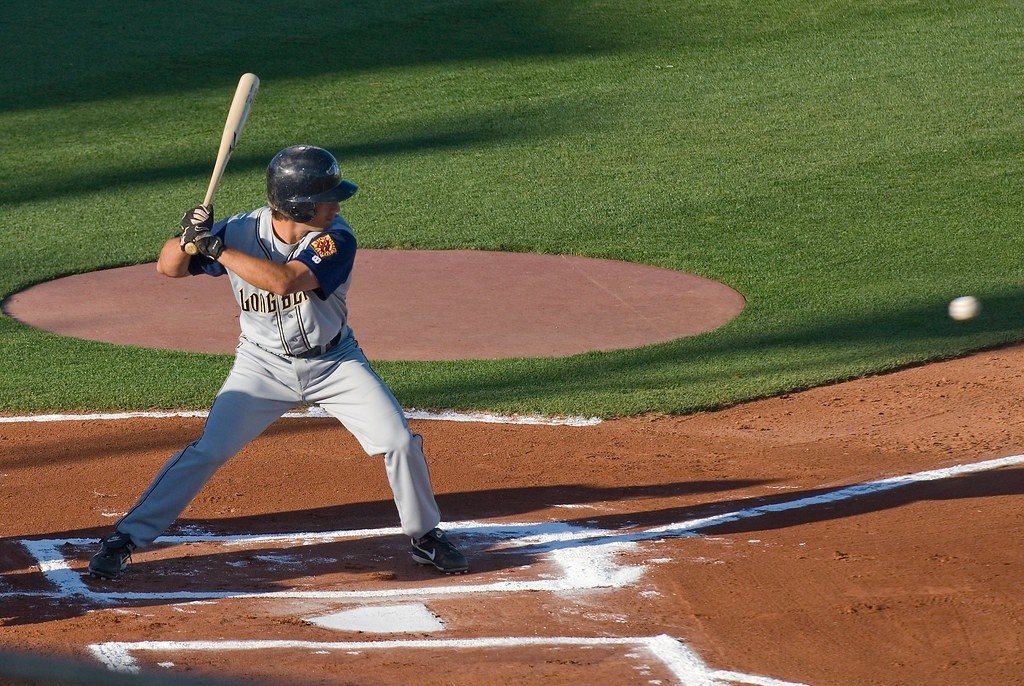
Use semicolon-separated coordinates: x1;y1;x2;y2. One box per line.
184;73;260;256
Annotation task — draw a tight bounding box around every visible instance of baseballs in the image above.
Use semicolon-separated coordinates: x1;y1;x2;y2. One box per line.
949;296;980;322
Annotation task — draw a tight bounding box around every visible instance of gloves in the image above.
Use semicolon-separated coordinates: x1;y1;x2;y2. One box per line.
179;203;214;233
180;225;226;261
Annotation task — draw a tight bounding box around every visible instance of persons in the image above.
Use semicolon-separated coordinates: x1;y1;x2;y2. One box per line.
88;143;469;583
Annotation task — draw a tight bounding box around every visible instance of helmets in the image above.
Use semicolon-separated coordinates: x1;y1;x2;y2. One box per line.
266;144;359;223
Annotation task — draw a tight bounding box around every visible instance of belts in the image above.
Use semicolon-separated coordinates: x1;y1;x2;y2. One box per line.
287;332;342;358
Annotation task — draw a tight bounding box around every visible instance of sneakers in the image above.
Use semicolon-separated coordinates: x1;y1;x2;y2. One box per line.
410;528;469;575
88;531;136;581
